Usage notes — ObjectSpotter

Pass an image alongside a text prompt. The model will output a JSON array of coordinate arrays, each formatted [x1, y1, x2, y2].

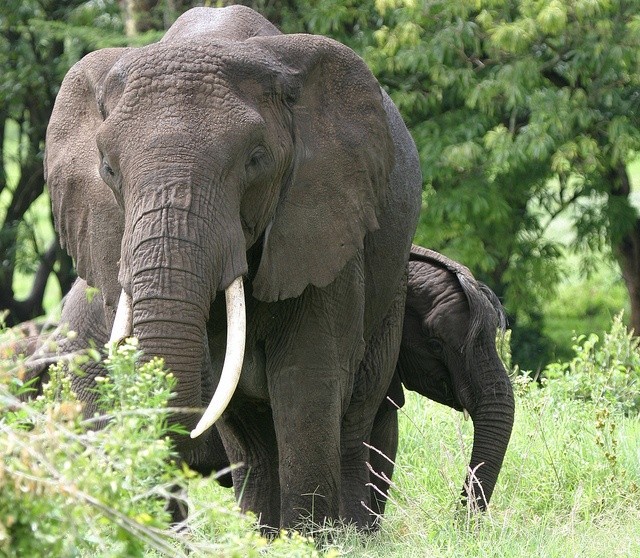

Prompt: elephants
[[7, 242, 516, 527], [42, 3, 424, 558]]
